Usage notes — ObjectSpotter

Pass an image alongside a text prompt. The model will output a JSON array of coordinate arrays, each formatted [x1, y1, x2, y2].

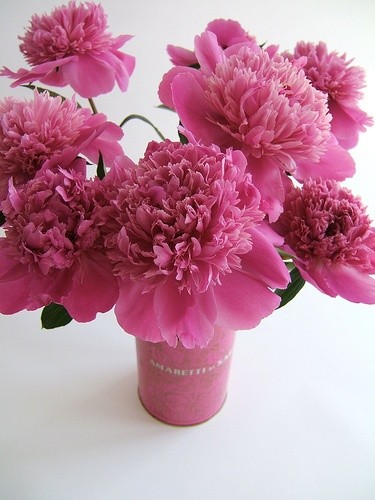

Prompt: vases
[[136, 325, 236, 426]]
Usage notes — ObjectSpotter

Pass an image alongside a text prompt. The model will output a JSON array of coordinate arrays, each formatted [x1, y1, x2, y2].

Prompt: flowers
[[0, 0, 374, 351]]
